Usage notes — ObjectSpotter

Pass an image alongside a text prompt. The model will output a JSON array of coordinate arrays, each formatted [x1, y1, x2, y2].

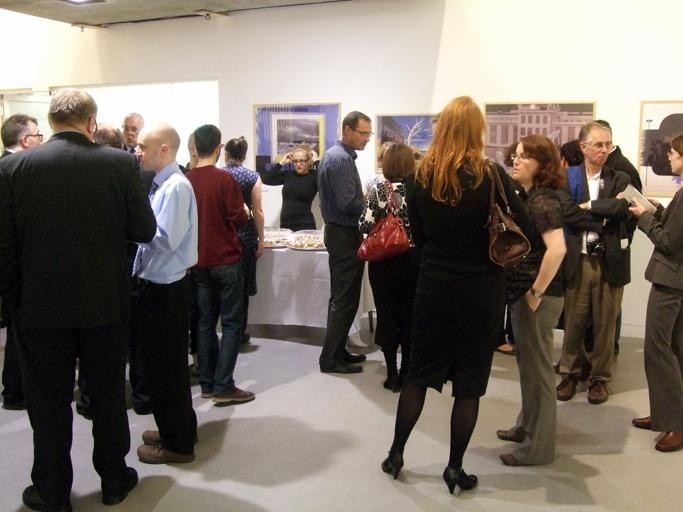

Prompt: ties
[[125, 146, 131, 153], [131, 183, 157, 278]]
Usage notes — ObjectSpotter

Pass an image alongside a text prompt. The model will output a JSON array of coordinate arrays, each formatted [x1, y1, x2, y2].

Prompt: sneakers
[[239, 334, 250, 344], [136, 444, 193, 463], [496, 342, 517, 356], [141, 431, 197, 445], [212, 387, 255, 402], [496, 430, 515, 441], [200, 382, 213, 398], [498, 453, 523, 465]]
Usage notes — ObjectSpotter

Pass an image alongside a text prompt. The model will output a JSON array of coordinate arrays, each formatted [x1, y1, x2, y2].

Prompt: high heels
[[442, 466, 477, 493], [381, 452, 403, 479], [383, 375, 401, 392]]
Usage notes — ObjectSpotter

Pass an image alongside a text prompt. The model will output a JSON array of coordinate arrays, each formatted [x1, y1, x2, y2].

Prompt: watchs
[[530, 287, 545, 299]]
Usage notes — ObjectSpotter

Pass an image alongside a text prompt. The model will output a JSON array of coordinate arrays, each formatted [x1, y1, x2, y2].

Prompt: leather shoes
[[631, 416, 652, 429], [318, 344, 362, 373], [655, 431, 683, 451], [21, 484, 72, 511], [555, 376, 576, 399], [76, 401, 94, 420], [101, 467, 137, 505], [343, 349, 366, 363], [587, 377, 613, 403]]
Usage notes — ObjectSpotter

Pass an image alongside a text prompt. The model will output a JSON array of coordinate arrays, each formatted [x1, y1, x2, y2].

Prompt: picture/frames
[[252, 103, 343, 173], [375, 113, 445, 174], [638, 100, 683, 198], [483, 102, 596, 175]]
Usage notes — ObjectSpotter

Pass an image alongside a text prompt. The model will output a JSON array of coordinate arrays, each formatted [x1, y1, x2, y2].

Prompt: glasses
[[24, 134, 42, 142], [353, 129, 375, 138], [509, 154, 541, 162], [582, 143, 616, 152], [120, 125, 138, 132], [290, 158, 308, 163], [666, 152, 678, 161], [88, 116, 97, 135]]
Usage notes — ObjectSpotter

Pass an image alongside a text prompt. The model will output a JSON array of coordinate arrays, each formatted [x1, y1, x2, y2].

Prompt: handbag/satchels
[[356, 213, 409, 262], [483, 200, 531, 271]]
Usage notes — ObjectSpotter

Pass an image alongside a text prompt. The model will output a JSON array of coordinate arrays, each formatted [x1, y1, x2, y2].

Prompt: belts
[[136, 278, 183, 287]]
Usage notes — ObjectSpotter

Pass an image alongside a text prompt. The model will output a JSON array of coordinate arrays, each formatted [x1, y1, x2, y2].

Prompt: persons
[[584, 119, 643, 363], [260, 144, 322, 231], [378, 93, 539, 494], [367, 141, 418, 394], [556, 120, 632, 404], [2, 89, 158, 509], [496, 141, 520, 354], [558, 139, 584, 168], [314, 109, 373, 374], [366, 142, 396, 190], [627, 130, 683, 453], [2, 113, 265, 464], [496, 135, 568, 465]]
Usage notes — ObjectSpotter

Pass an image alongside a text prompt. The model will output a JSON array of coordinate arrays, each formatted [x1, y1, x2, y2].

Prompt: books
[[621, 183, 658, 219]]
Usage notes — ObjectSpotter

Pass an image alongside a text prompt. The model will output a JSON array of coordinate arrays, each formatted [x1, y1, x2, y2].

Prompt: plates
[[286, 235, 328, 251]]
